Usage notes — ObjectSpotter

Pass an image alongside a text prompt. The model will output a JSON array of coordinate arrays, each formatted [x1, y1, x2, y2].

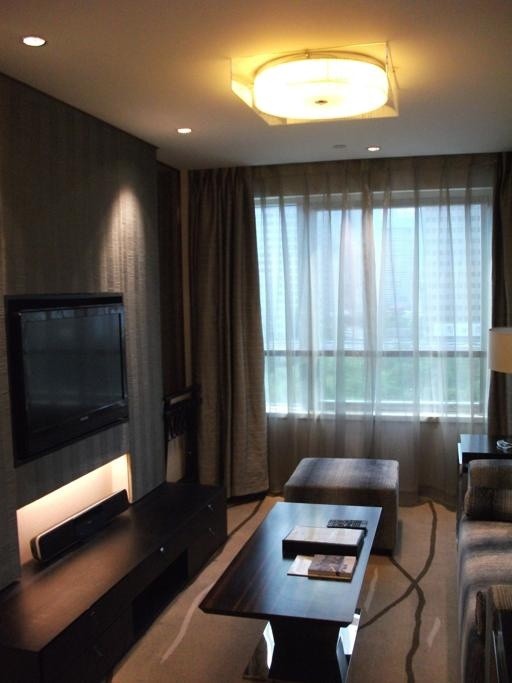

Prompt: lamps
[[487, 326, 512, 454], [231, 40, 399, 126]]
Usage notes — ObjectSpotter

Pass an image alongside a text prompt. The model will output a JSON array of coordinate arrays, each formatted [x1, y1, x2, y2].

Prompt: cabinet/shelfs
[[1, 482, 227, 682]]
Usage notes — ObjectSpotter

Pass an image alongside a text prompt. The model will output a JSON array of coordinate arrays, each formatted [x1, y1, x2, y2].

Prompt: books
[[306, 553, 357, 578], [286, 552, 358, 581]]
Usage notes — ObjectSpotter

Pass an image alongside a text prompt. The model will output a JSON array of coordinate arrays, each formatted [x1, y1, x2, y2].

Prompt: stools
[[283, 457, 399, 557]]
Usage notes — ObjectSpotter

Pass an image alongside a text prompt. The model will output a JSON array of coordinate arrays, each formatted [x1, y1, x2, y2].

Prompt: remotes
[[327, 519, 368, 528]]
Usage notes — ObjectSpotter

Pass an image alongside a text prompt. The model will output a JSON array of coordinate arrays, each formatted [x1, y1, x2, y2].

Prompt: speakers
[[29, 488, 130, 562]]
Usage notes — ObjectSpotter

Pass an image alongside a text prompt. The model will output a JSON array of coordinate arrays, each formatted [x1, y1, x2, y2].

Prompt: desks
[[457, 434, 511, 476]]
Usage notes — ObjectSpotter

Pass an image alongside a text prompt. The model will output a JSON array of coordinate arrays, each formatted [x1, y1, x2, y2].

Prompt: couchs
[[456, 458, 511, 682]]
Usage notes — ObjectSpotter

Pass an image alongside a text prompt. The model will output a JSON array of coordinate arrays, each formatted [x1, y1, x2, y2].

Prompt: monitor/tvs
[[4, 292, 131, 468]]
